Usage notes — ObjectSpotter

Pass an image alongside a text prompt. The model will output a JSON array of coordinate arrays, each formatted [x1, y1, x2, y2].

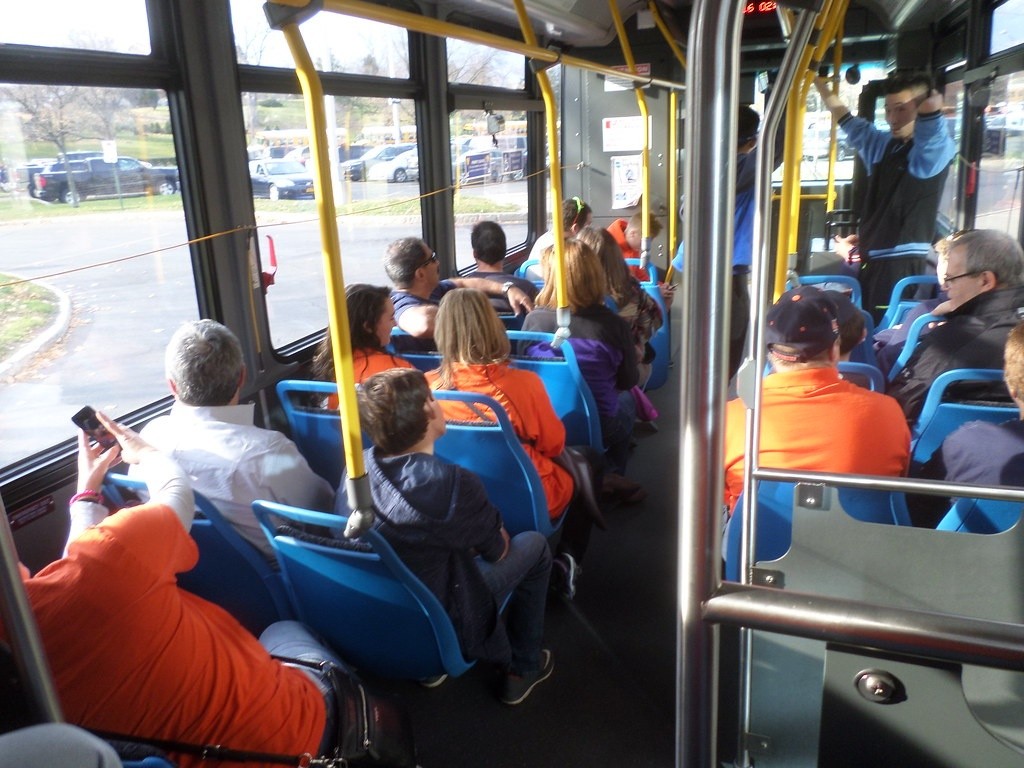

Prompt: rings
[[520, 297, 526, 303]]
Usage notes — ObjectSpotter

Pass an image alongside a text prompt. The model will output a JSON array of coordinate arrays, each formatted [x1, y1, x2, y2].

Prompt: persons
[[831, 235, 862, 277], [125, 320, 335, 573], [425, 288, 605, 601], [721, 284, 911, 569], [308, 283, 416, 408], [875, 229, 1024, 531], [517, 237, 648, 502], [16, 412, 354, 767], [825, 291, 867, 361], [468, 221, 538, 300], [329, 367, 574, 705], [729, 71, 787, 375], [384, 238, 534, 338], [811, 70, 955, 324], [529, 194, 677, 432]]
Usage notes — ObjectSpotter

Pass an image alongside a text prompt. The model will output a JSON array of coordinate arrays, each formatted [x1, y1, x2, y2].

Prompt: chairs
[[0, 259, 1024, 767]]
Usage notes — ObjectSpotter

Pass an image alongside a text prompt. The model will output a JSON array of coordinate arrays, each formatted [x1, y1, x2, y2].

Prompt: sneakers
[[502, 649, 554, 706]]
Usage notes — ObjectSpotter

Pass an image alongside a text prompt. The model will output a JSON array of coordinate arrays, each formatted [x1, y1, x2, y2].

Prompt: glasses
[[935, 270, 1000, 293], [573, 196, 587, 226], [420, 251, 439, 269]]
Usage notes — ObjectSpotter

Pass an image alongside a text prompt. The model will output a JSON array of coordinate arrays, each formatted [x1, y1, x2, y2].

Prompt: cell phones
[[71, 406, 123, 457]]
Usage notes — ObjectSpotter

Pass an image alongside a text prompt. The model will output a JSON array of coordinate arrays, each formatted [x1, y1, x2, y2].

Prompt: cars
[[804, 122, 856, 162], [0, 133, 529, 209]]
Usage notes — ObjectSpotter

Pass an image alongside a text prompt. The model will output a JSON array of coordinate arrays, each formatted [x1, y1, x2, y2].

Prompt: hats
[[825, 290, 863, 357], [768, 286, 843, 362]]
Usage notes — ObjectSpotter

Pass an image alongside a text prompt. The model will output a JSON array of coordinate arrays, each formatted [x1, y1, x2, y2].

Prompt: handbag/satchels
[[320, 660, 416, 768], [630, 384, 660, 422]]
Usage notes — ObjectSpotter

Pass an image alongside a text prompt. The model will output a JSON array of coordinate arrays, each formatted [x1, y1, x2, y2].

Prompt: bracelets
[[69, 490, 104, 503], [502, 282, 515, 298]]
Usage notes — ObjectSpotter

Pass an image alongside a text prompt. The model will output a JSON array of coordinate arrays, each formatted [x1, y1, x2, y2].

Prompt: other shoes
[[602, 473, 640, 499], [574, 564, 583, 577], [614, 491, 650, 506], [553, 552, 576, 602], [633, 417, 658, 434], [421, 673, 448, 688]]
